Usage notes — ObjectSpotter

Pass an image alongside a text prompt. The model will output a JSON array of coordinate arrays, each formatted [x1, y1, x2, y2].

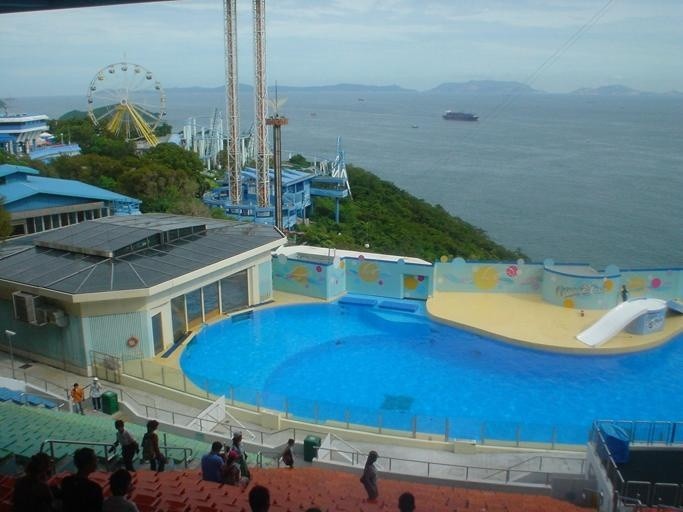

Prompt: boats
[[442, 109, 480, 121]]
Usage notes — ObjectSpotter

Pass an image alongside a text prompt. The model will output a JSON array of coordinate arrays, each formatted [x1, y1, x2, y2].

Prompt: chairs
[[0, 386, 682, 512]]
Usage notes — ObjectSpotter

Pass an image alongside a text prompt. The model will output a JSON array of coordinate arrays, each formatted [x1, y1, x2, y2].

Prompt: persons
[[399, 492, 415, 511], [621, 285, 629, 302], [363, 451, 378, 499], [112, 420, 138, 473], [13, 451, 53, 511], [89, 377, 103, 411], [71, 382, 85, 414], [249, 485, 270, 512], [103, 469, 139, 511], [142, 420, 165, 471], [61, 447, 104, 511], [201, 431, 250, 492], [283, 438, 295, 467]]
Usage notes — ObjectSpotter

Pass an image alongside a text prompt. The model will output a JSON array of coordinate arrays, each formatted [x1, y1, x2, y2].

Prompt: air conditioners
[[11, 291, 69, 328]]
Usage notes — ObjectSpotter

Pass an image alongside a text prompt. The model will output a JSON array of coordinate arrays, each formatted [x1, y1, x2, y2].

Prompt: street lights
[[263, 82, 290, 233], [6, 329, 16, 380], [60, 133, 63, 144]]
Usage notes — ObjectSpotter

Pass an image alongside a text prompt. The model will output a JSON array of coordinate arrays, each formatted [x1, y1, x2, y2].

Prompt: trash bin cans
[[303, 435, 321, 462], [101, 391, 118, 415]]
